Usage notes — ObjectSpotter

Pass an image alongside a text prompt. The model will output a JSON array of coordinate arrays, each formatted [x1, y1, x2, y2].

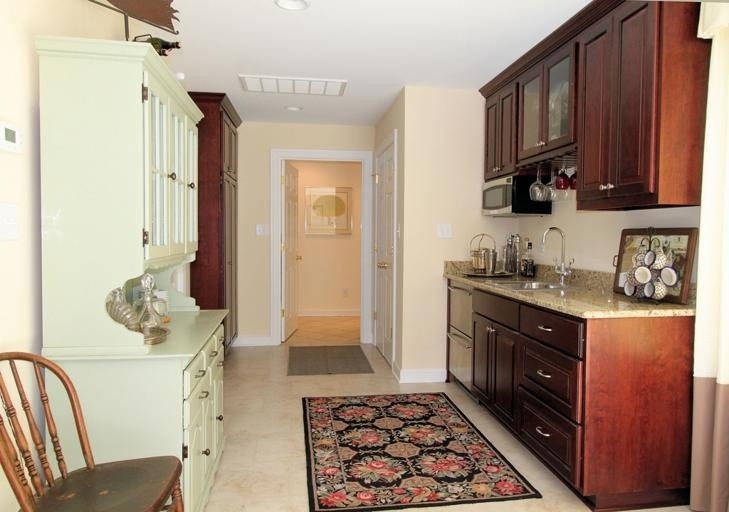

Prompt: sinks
[[491, 280, 565, 292]]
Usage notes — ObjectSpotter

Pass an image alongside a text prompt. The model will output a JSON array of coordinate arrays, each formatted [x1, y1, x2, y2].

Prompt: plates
[[463, 271, 514, 278]]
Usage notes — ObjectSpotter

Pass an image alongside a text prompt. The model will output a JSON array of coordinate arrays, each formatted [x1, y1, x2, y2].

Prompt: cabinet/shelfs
[[519, 294, 694, 512], [480, 60, 518, 183], [186, 93, 242, 352], [39, 32, 231, 512], [517, 13, 578, 170], [575, 1, 712, 211], [472, 287, 519, 443]]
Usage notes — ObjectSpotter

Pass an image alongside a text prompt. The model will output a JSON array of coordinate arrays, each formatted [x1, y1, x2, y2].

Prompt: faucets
[[542, 226, 575, 286]]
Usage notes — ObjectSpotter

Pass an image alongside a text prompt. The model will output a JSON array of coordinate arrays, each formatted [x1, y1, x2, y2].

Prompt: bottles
[[106, 288, 138, 333], [522, 238, 529, 275], [514, 234, 521, 274], [138, 272, 163, 338], [506, 234, 512, 272], [526, 242, 535, 277]]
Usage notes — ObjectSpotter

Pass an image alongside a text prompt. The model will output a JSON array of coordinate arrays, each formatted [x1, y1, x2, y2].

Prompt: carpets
[[301, 391, 542, 512], [287, 345, 374, 378]]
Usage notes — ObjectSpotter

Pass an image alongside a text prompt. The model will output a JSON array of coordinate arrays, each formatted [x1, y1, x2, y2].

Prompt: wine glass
[[556, 162, 571, 189], [555, 159, 568, 202], [568, 162, 578, 189], [547, 163, 558, 201], [529, 162, 548, 203]]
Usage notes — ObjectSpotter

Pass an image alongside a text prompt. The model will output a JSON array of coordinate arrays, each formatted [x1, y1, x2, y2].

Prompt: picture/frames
[[303, 185, 355, 235], [612, 223, 698, 306]]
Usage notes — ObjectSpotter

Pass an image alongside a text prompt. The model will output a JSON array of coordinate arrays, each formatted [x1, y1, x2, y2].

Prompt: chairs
[[0, 353, 186, 512]]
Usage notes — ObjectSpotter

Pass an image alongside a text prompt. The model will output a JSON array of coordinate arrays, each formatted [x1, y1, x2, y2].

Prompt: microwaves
[[481, 175, 552, 215]]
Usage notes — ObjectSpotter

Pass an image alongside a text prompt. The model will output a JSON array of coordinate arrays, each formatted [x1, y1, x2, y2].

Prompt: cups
[[626, 266, 652, 287], [631, 247, 646, 268], [655, 266, 678, 286], [483, 249, 497, 274], [624, 280, 644, 299], [644, 246, 667, 270], [644, 277, 667, 300]]
